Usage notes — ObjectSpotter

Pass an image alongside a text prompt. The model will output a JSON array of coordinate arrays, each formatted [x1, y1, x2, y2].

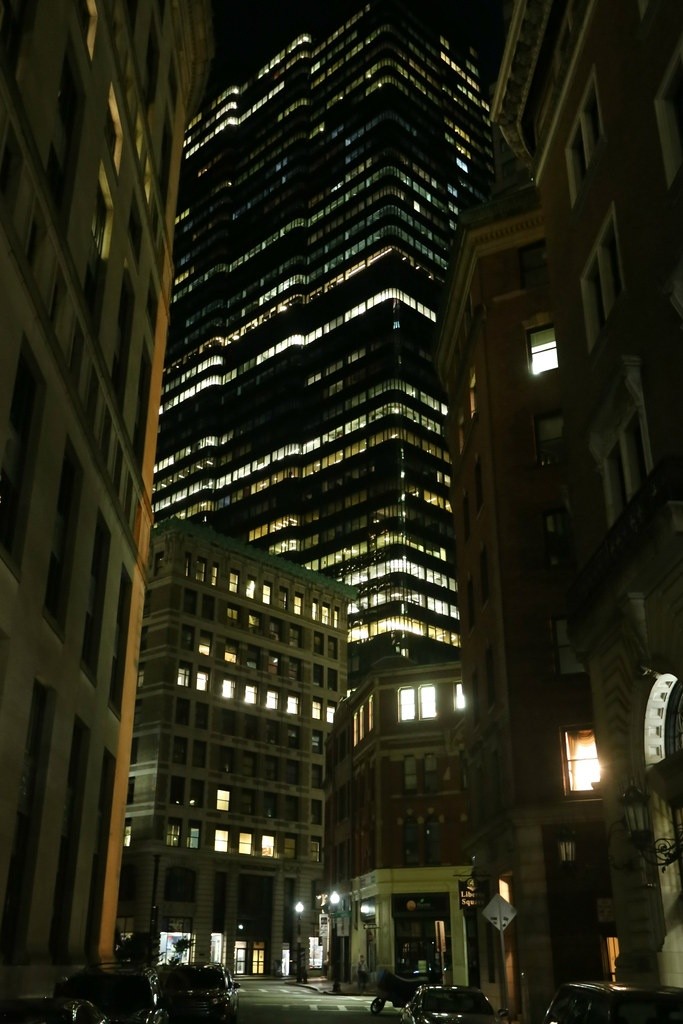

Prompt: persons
[[355, 953, 368, 995]]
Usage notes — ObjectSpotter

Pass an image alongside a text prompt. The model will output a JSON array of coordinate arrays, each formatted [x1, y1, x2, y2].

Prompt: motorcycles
[[372, 970, 431, 1012]]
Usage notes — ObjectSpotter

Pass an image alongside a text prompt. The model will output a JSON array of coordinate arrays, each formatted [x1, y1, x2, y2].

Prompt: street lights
[[328, 890, 345, 991], [295, 900, 304, 982]]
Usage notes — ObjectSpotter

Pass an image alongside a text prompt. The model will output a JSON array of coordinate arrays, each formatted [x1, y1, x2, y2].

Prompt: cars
[[0, 995, 111, 1024], [399, 984, 509, 1024]]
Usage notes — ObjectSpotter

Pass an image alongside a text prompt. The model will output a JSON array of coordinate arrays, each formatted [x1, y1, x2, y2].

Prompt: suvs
[[55, 961, 169, 1024], [164, 961, 241, 1024], [541, 982, 683, 1024]]
